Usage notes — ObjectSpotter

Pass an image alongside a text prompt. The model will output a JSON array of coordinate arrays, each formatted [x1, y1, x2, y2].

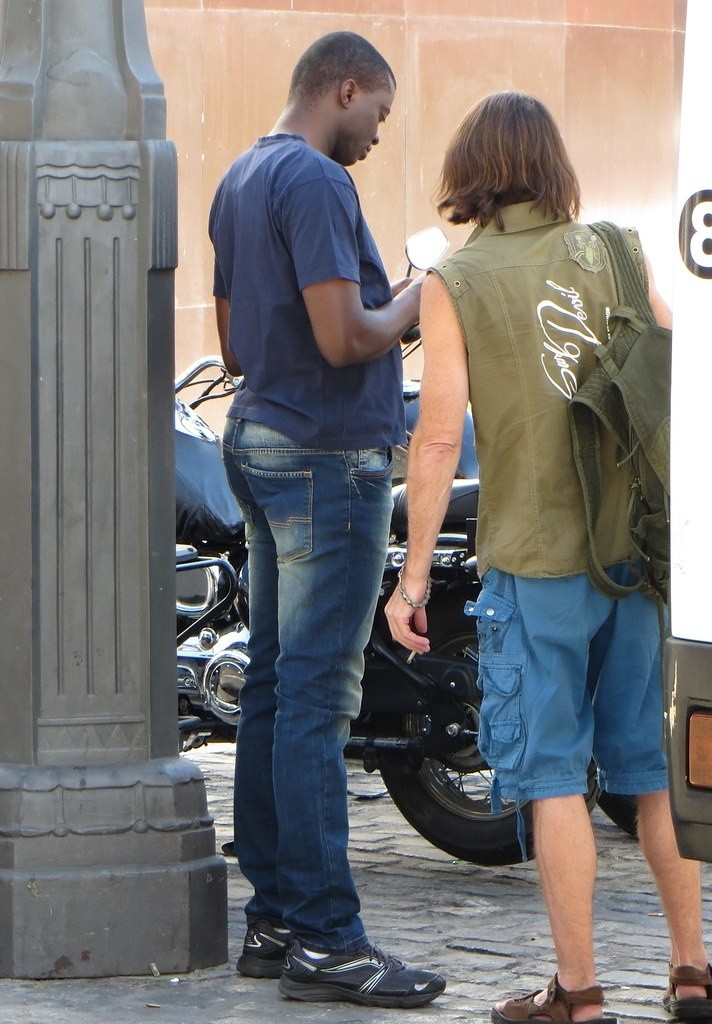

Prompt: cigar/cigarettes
[[407, 649, 416, 664]]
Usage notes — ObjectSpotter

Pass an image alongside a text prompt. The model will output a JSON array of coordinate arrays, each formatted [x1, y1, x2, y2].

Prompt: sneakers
[[236, 920, 296, 977], [279, 938, 446, 1008]]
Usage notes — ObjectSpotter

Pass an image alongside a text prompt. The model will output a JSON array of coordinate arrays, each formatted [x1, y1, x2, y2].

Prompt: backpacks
[[568, 220, 673, 750]]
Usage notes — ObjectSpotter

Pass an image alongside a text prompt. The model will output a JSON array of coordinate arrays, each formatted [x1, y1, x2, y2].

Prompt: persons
[[385, 92, 711, 1023], [208, 27, 448, 1007]]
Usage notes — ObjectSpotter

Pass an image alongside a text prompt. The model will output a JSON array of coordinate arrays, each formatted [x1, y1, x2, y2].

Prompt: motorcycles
[[172, 225, 640, 866]]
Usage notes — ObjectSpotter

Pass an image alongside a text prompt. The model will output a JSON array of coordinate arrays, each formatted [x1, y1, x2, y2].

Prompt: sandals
[[491, 972, 618, 1024], [662, 962, 712, 1018]]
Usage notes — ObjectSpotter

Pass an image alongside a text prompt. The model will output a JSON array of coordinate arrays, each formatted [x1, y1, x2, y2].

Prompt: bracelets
[[397, 565, 433, 608]]
